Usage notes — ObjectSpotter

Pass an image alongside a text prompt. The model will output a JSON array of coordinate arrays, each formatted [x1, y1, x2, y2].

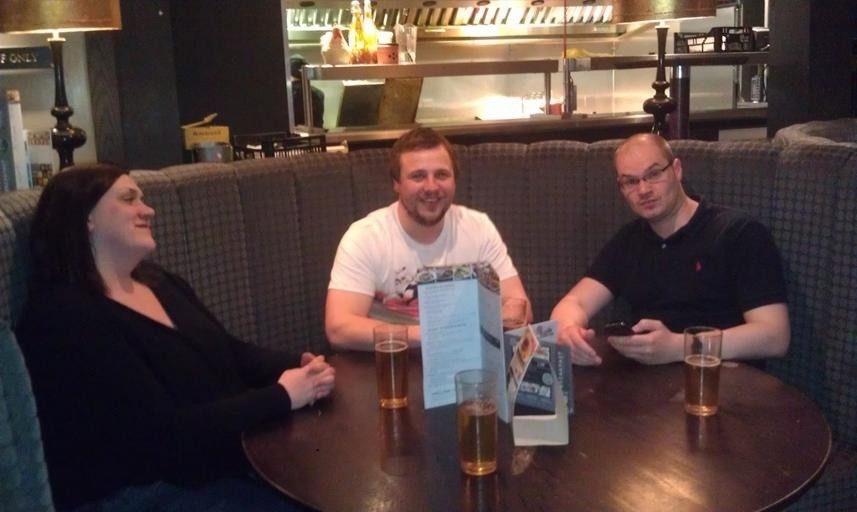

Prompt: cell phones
[[602, 321, 632, 338]]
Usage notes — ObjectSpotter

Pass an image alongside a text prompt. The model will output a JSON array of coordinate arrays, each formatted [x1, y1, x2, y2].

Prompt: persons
[[320, 125, 535, 353], [25, 163, 338, 511], [548, 131, 792, 367], [289, 53, 324, 127]]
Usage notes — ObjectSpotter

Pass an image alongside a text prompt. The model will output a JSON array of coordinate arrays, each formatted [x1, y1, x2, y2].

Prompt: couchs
[[0, 141, 849, 511]]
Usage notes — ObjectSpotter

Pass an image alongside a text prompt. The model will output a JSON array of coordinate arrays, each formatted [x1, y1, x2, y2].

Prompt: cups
[[682, 325, 723, 417], [372, 322, 409, 409], [455, 368, 499, 476], [394, 23, 418, 63]]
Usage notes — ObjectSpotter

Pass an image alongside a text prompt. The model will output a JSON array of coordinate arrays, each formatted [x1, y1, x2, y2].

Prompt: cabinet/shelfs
[[297, 57, 782, 150]]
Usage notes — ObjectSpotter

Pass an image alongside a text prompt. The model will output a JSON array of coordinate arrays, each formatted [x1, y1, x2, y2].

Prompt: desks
[[239, 329, 834, 512]]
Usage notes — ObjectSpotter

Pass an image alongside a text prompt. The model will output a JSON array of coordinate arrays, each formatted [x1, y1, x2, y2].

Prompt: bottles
[[347, 0, 379, 62]]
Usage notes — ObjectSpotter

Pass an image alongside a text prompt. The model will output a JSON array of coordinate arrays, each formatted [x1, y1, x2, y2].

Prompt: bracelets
[[689, 332, 702, 355]]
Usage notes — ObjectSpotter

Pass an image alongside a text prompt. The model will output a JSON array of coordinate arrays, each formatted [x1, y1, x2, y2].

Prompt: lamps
[[0, 0, 122, 169]]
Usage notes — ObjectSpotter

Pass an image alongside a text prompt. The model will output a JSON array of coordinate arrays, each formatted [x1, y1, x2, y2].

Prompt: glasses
[[616, 161, 673, 191]]
